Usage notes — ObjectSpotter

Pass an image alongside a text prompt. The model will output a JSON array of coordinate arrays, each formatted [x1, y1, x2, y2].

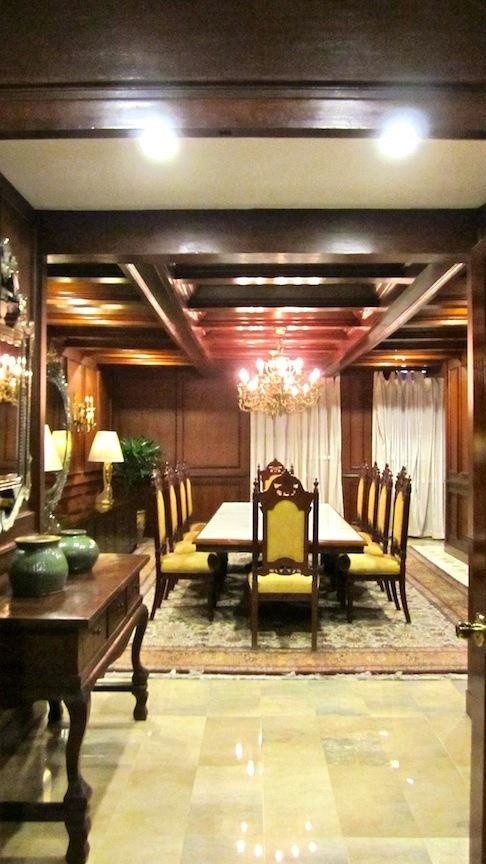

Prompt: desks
[[0, 553, 149, 864]]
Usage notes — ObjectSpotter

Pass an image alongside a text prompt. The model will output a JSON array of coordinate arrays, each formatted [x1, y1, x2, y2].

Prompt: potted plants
[[112, 434, 161, 541]]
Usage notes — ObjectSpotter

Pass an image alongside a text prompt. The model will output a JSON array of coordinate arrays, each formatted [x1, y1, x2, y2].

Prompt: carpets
[[105, 538, 469, 673]]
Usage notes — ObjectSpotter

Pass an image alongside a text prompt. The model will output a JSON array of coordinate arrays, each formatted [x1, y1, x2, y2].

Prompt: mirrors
[[0, 237, 35, 531], [45, 342, 72, 532]]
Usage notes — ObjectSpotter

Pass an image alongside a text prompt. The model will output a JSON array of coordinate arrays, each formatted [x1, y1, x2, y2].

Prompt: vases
[[7, 535, 69, 598], [58, 528, 99, 573]]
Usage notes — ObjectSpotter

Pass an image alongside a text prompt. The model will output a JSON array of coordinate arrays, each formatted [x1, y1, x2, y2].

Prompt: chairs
[[352, 461, 380, 539], [248, 478, 326, 650], [336, 466, 410, 624], [150, 461, 221, 624], [257, 460, 295, 497], [335, 464, 394, 599]]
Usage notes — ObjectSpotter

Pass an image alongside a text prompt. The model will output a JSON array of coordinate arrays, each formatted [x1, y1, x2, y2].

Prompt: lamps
[[87, 430, 124, 505], [73, 396, 96, 433], [44, 424, 63, 472], [0, 353, 32, 407], [51, 430, 71, 466], [236, 330, 322, 424]]
